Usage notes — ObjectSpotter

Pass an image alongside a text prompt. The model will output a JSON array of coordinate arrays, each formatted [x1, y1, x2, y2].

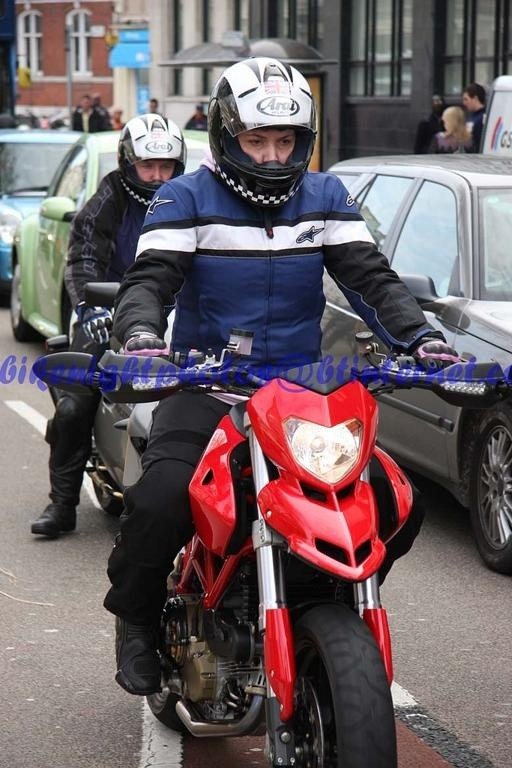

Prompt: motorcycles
[[33, 328, 512, 765], [42, 280, 177, 522]]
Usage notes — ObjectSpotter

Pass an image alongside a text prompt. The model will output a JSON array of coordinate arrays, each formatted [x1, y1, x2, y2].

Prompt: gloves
[[412, 337, 460, 374], [123, 323, 170, 356], [78, 304, 113, 344]]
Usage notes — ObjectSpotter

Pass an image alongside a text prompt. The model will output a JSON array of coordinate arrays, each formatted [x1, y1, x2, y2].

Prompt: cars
[[7, 124, 213, 355], [314, 148, 511, 577], [1, 125, 87, 304]]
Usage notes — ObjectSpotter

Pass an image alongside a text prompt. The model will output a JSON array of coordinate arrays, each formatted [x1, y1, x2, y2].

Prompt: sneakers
[[116, 614, 160, 692], [32, 502, 75, 533]]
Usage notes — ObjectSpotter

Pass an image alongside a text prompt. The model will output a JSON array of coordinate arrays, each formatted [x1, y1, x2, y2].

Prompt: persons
[[1, 94, 210, 132], [31, 113, 187, 539], [462, 83, 488, 154], [103, 56, 461, 696], [427, 106, 474, 154]]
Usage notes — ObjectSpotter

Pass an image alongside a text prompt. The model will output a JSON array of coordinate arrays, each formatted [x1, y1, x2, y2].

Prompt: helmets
[[207, 55, 316, 209], [118, 113, 187, 209]]
